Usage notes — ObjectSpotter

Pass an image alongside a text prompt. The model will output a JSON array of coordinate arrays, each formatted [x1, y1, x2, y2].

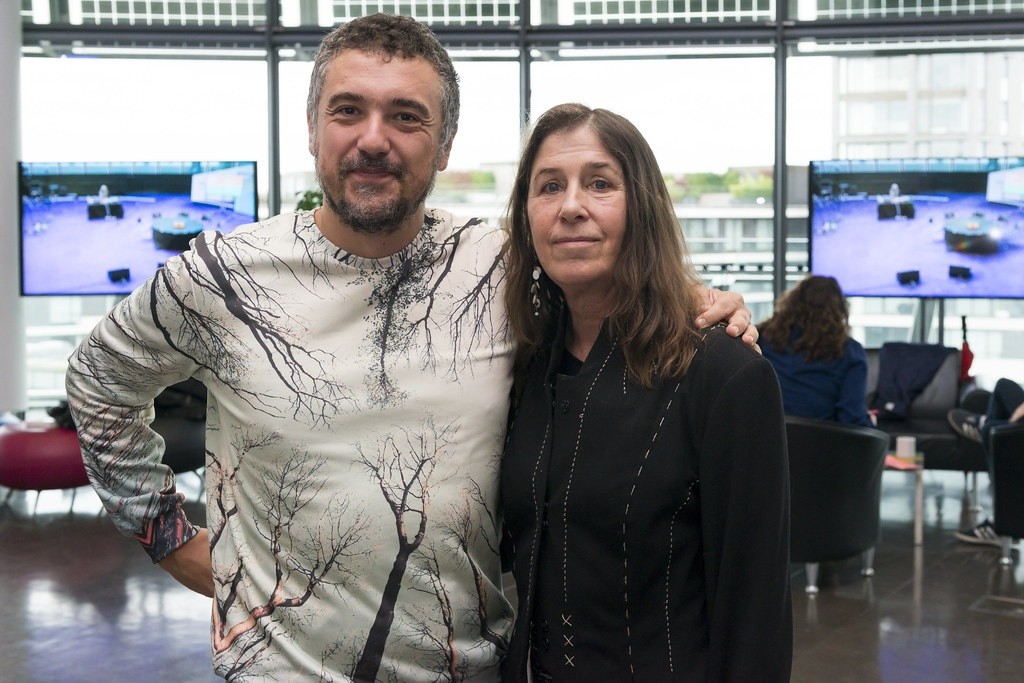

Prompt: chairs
[[863, 347, 1024, 547], [787, 414, 891, 592]]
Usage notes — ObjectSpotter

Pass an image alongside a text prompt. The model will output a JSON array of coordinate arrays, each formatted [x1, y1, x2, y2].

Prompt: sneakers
[[954, 519, 1020, 548], [947, 408, 989, 444]]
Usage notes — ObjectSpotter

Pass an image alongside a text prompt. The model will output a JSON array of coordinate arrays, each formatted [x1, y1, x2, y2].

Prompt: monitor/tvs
[[18, 161, 258, 297], [808, 156, 1024, 300]]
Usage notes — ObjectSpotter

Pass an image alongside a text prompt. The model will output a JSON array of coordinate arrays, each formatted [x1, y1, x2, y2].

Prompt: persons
[[947, 378, 1024, 545], [65, 13, 762, 683], [500, 103, 793, 683], [756, 275, 875, 428]]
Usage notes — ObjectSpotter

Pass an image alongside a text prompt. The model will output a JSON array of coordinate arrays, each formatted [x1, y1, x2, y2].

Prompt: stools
[[0, 417, 207, 518]]
